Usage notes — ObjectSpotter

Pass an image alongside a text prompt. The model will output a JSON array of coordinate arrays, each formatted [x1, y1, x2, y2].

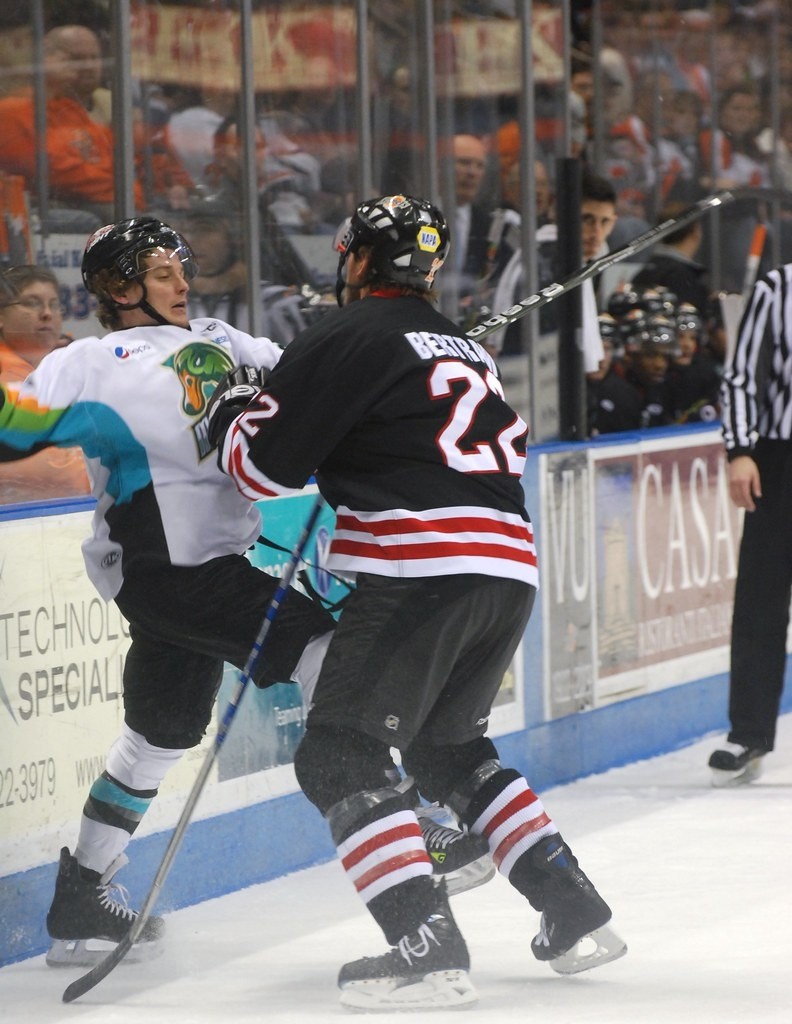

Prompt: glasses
[[0, 298, 67, 316]]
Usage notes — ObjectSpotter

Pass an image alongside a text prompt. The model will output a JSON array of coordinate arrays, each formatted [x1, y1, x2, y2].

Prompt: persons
[[488, 178, 619, 373], [4, 216, 333, 964], [7, 20, 189, 219], [452, 132, 495, 273], [703, 257, 790, 786], [493, 4, 791, 434], [209, 196, 628, 1006], [4, 260, 68, 406]]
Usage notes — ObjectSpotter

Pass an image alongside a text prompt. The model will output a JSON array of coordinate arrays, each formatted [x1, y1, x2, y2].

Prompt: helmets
[[350, 194, 450, 289], [593, 282, 702, 354], [82, 216, 170, 294]]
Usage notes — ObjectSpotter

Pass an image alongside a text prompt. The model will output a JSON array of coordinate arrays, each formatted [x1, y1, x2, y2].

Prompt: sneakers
[[506, 834, 628, 975], [709, 732, 774, 788], [46, 846, 166, 962], [337, 874, 478, 1008], [394, 776, 496, 896]]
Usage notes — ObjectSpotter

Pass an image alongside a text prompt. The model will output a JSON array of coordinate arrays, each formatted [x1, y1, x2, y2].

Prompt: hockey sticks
[[467, 190, 734, 345], [60, 491, 328, 1004]]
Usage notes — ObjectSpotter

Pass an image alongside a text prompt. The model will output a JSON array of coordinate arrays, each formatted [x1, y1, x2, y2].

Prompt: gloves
[[206, 363, 272, 476]]
